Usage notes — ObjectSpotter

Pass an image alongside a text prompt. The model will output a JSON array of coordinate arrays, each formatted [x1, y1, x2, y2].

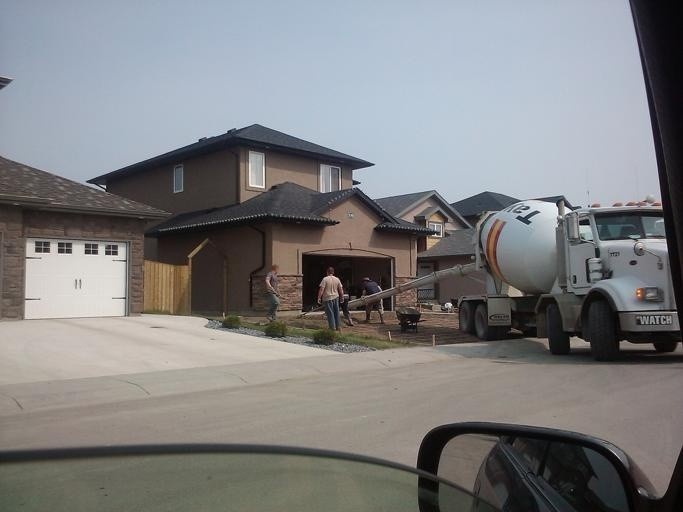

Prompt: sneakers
[[267, 315, 276, 322], [327, 326, 342, 331]]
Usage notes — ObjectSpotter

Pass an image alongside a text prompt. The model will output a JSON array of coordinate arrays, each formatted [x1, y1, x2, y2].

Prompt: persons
[[318, 267, 345, 333], [334, 272, 354, 326], [360, 275, 385, 323], [263, 264, 282, 322]]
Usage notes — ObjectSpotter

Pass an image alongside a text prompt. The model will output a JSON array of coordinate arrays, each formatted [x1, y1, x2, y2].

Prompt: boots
[[363, 314, 371, 323], [347, 317, 354, 326], [380, 314, 386, 323]]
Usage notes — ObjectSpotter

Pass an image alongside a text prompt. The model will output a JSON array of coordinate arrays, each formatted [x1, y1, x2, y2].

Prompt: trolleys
[[395, 306, 420, 333]]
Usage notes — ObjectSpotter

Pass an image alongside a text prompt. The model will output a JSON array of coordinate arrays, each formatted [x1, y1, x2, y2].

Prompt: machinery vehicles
[[456, 197, 681, 362]]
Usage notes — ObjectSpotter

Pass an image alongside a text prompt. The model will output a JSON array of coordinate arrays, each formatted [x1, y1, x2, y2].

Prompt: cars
[[471, 434, 630, 511]]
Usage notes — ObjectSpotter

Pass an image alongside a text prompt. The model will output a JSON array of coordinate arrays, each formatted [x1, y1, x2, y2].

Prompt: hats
[[360, 277, 373, 282]]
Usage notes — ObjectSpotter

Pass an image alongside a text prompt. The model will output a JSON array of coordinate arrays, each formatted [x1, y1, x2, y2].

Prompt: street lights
[[0, 75, 14, 90]]
